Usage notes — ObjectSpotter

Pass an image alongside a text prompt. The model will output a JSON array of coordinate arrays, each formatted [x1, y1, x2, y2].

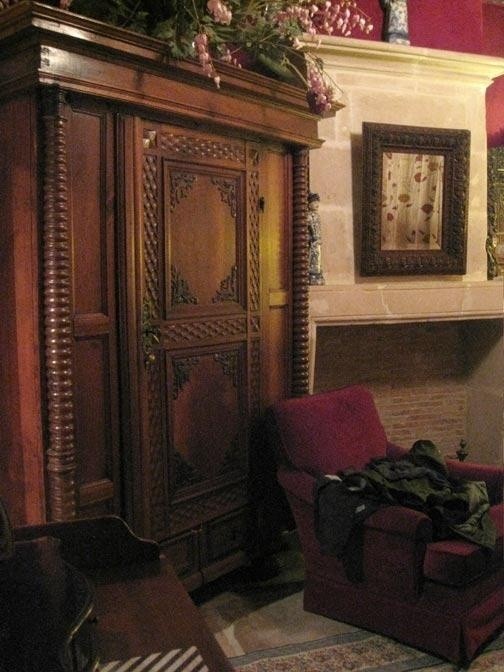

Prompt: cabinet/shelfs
[[0, 0, 348, 592]]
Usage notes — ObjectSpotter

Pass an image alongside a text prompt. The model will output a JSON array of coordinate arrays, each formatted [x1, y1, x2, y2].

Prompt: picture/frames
[[361, 120, 470, 276]]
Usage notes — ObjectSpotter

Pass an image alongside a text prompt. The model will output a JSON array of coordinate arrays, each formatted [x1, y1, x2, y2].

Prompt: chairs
[[274, 440, 504, 670]]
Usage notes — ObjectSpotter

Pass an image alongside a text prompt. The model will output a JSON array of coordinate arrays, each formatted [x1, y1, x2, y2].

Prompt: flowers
[[57, 0, 374, 117]]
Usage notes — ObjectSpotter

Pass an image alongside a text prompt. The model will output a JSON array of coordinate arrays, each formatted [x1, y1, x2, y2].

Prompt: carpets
[[226, 622, 504, 672]]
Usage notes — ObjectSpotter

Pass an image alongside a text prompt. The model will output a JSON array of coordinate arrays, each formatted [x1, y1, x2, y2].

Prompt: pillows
[[276, 385, 387, 476]]
